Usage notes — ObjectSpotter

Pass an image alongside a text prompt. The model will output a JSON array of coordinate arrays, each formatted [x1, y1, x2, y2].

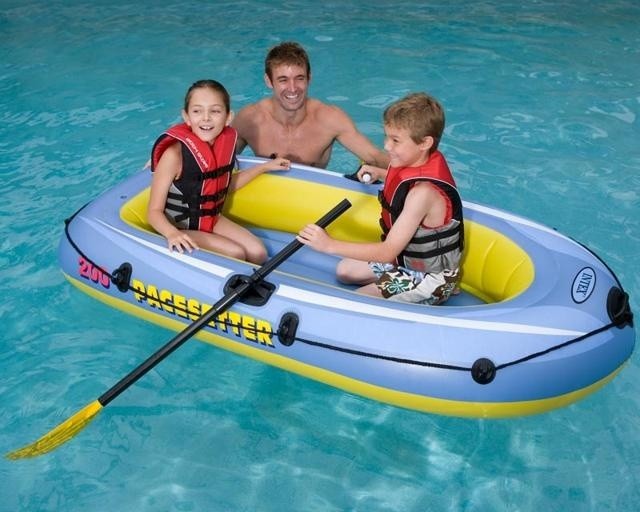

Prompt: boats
[[57, 155, 636, 418]]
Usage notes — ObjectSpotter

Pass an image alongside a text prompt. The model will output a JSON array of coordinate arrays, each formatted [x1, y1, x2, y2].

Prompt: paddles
[[6, 198, 351, 462]]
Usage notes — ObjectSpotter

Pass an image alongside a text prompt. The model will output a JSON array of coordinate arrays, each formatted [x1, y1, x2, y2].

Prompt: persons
[[295, 90, 465, 308], [227, 38, 393, 176], [147, 79, 291, 269]]
